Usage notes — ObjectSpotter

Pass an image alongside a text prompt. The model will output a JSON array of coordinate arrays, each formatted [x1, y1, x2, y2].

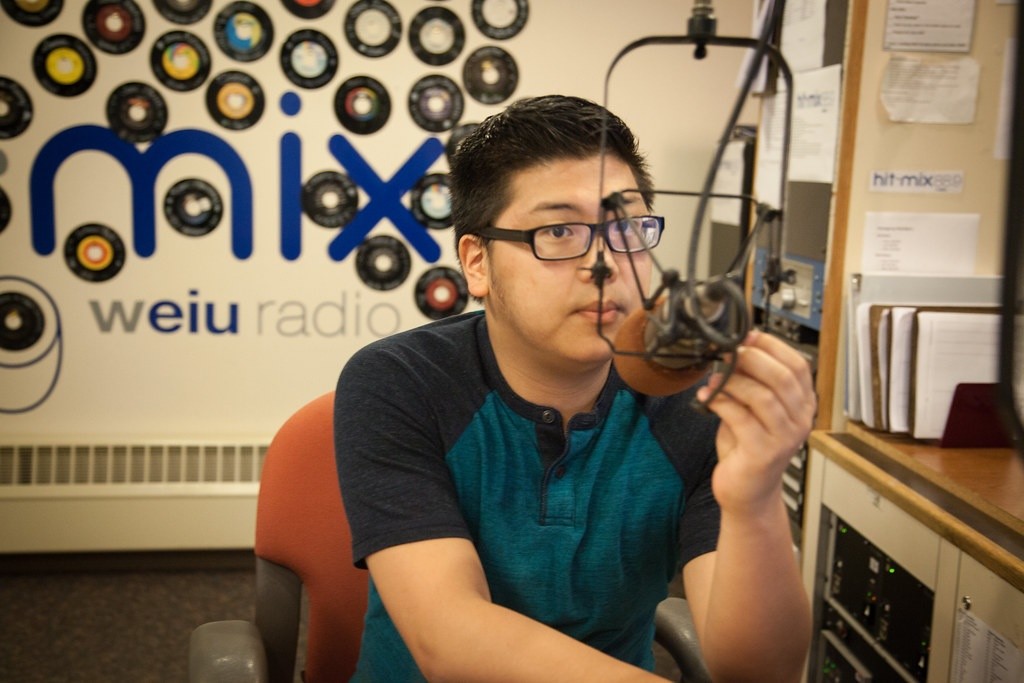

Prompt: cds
[[0, 0, 529, 353]]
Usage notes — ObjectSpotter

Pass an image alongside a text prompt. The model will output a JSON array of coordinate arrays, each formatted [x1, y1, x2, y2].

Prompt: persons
[[333, 94, 816, 683]]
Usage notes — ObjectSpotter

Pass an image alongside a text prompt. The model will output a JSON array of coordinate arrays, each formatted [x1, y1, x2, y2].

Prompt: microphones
[[613, 275, 732, 397]]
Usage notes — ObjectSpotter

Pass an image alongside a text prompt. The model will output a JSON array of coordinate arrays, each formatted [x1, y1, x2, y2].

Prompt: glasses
[[471, 215, 665, 261]]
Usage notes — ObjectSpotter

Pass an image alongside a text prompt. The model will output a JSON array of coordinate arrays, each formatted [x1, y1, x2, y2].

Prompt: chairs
[[188, 386, 381, 683]]
